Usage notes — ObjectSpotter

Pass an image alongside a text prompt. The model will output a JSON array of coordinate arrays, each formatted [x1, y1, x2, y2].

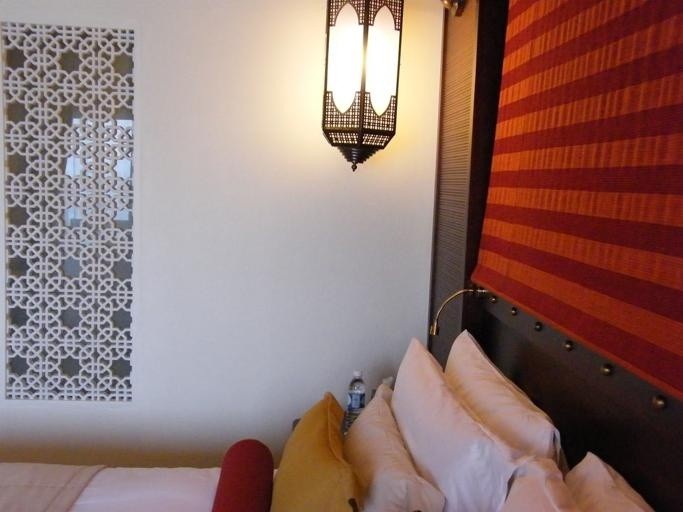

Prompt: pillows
[[496, 454, 581, 511], [444, 328, 565, 463], [564, 449, 656, 511], [338, 378, 446, 511], [268, 390, 369, 512], [210, 439, 275, 512], [391, 336, 538, 511]]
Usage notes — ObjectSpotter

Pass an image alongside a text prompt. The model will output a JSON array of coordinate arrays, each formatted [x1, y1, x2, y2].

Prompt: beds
[[0, 326, 683, 512]]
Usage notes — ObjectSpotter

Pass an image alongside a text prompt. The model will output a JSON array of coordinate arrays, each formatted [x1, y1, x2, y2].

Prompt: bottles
[[345, 370, 366, 429]]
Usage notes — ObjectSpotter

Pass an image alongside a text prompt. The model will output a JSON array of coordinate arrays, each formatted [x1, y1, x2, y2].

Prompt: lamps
[[319, 0, 466, 173], [429, 286, 487, 335]]
